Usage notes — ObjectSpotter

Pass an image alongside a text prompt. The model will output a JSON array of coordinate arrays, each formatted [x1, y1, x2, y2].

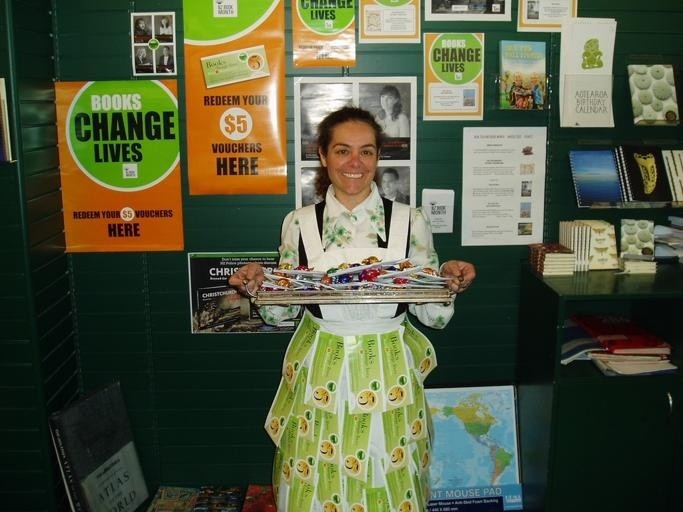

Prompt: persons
[[134, 47, 150, 66], [159, 16, 172, 36], [373, 86, 409, 138], [133, 17, 146, 37], [226, 107, 477, 512], [378, 168, 409, 205], [158, 45, 174, 73]]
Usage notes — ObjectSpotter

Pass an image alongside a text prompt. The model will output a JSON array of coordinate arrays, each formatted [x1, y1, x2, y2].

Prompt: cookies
[[628, 65, 679, 125], [621, 219, 653, 256]]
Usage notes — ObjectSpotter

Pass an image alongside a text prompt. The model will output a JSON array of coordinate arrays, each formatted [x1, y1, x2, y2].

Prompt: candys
[[265, 256, 438, 291]]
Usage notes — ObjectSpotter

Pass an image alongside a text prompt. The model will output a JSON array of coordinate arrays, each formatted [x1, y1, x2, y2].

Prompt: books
[[567, 148, 683, 210], [525, 216, 683, 277], [556, 313, 678, 376]]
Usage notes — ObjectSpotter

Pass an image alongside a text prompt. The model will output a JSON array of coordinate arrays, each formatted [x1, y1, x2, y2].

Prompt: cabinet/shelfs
[[517, 260, 681, 512]]
